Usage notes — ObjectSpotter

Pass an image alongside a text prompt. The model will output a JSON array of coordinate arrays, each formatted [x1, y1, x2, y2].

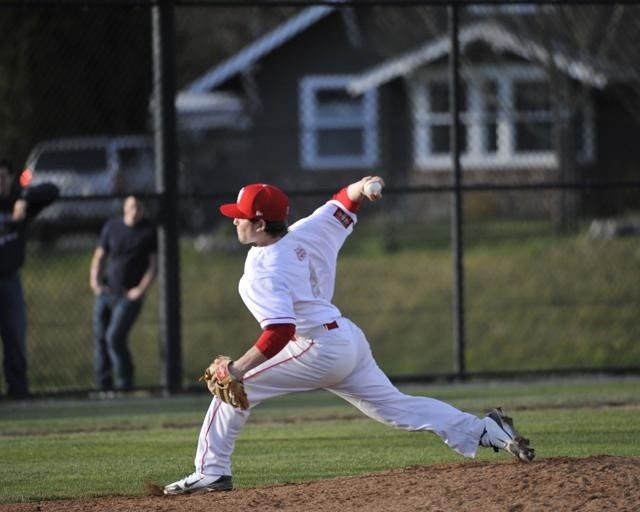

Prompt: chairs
[[479, 406, 536, 463], [164, 472, 232, 495]]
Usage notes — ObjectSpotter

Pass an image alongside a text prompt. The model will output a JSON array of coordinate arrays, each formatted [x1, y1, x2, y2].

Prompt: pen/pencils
[[21, 138, 199, 251]]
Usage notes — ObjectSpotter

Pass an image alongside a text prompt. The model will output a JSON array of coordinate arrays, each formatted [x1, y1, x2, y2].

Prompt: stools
[[291, 320, 338, 341]]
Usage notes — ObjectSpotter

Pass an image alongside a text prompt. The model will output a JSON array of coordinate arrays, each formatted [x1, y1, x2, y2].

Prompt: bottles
[[220, 183, 290, 222]]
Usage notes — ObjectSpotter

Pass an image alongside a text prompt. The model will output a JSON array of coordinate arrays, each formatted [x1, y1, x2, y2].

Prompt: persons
[[85, 192, 158, 399], [0, 157, 35, 399], [157, 175, 536, 494]]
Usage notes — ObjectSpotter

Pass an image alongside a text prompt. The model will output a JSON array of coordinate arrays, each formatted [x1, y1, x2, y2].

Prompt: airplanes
[[205, 356, 251, 413]]
